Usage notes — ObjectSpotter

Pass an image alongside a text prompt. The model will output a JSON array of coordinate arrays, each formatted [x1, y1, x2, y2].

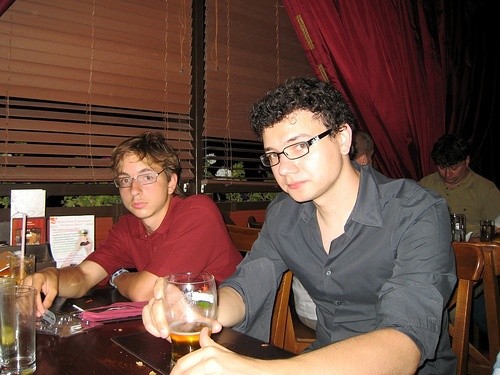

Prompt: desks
[[0, 269, 299, 375]]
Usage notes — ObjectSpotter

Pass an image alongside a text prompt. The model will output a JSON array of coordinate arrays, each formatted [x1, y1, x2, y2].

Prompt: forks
[[72, 304, 121, 312]]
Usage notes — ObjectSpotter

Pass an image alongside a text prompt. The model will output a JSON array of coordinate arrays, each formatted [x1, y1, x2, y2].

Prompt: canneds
[[450, 214, 466, 242]]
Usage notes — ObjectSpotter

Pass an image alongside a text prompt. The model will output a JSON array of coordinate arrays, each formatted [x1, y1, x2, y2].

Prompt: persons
[[17, 131, 243, 318], [141, 74, 457, 375], [417, 135, 500, 332], [292, 132, 374, 329]]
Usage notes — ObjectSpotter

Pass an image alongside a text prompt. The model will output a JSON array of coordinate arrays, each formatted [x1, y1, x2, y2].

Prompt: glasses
[[260, 128, 333, 168], [113, 167, 168, 188]]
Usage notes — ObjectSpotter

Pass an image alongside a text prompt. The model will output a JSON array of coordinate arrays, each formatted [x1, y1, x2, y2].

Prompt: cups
[[450, 213, 466, 243], [163, 271, 218, 372], [0, 285, 37, 375], [480, 218, 495, 242], [9, 252, 36, 283]]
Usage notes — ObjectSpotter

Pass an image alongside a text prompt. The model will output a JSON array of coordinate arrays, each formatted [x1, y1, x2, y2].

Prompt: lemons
[[1, 326, 15, 345]]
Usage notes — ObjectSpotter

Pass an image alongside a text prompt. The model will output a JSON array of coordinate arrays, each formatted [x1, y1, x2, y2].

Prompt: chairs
[[228, 228, 500, 375]]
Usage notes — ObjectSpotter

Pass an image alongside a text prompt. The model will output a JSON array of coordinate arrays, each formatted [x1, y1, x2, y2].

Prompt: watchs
[[110, 268, 129, 288]]
